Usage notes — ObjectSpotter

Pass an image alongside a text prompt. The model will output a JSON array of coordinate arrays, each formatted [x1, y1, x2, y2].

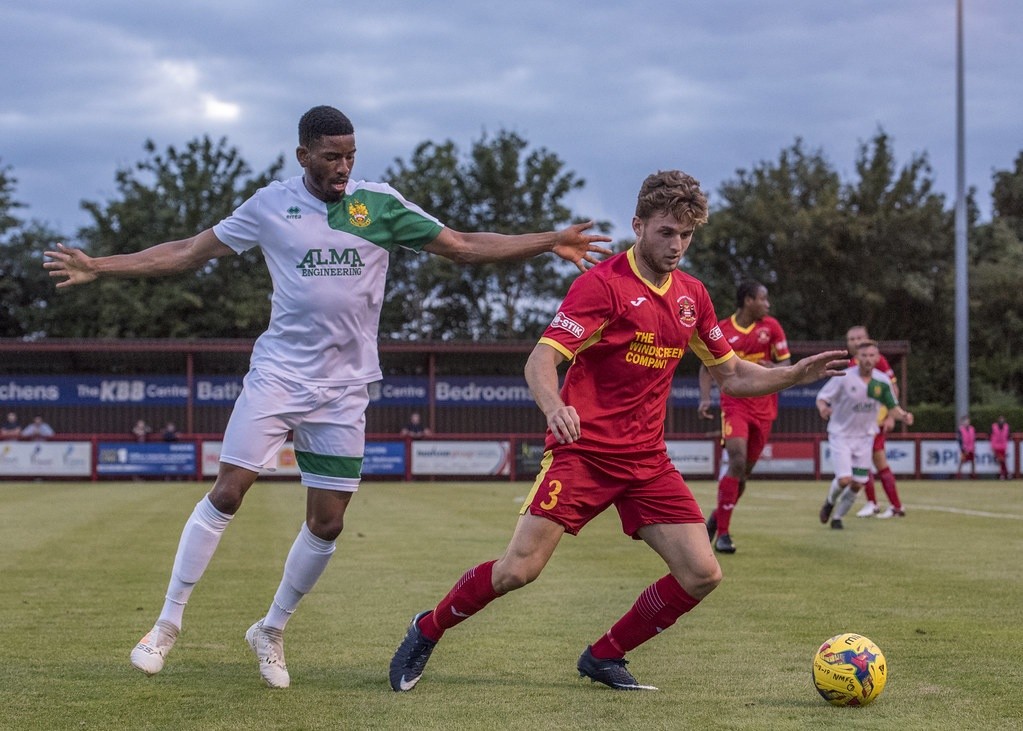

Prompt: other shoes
[[820, 498, 837, 523], [876, 505, 905, 519], [706, 514, 717, 543], [856, 500, 880, 518], [715, 535, 735, 553], [831, 519, 841, 529]]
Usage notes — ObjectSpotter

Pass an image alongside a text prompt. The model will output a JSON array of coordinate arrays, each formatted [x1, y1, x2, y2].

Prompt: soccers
[[812, 632, 888, 705]]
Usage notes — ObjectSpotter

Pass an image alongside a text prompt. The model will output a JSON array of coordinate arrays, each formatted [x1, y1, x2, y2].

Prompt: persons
[[23, 416, 53, 436], [956, 416, 977, 480], [43, 106, 612, 689], [164, 422, 180, 440], [133, 420, 151, 435], [402, 413, 430, 434], [697, 280, 792, 553], [0, 413, 21, 436], [989, 415, 1012, 480], [848, 324, 906, 520], [816, 341, 913, 530], [388, 170, 851, 692]]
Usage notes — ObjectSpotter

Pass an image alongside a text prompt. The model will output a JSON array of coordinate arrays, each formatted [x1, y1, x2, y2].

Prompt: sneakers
[[576, 645, 657, 690], [244, 619, 291, 690], [390, 610, 439, 692], [130, 625, 175, 677]]
